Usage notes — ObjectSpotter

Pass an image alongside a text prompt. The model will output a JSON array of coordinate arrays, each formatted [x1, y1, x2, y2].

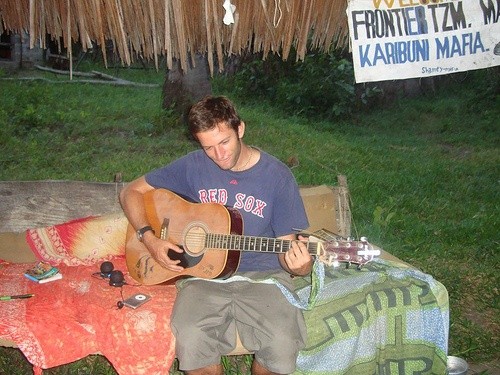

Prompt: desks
[[0, 173, 449, 375]]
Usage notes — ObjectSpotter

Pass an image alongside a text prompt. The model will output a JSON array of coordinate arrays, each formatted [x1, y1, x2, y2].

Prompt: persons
[[118, 97, 316, 375]]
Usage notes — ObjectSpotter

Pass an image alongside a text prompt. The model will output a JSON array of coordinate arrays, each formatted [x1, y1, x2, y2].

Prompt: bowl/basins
[[447, 356, 469, 375]]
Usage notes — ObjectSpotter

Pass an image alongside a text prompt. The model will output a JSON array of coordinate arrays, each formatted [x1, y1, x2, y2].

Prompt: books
[[22, 261, 63, 284]]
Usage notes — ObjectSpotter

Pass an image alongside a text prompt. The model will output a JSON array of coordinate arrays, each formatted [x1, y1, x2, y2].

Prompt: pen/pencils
[[0, 294, 35, 300]]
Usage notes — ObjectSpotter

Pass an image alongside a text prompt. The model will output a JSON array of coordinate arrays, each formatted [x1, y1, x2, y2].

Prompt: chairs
[[298, 175, 352, 238]]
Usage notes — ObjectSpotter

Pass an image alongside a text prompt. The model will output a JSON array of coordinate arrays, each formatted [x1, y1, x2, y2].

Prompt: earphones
[[117, 301, 123, 308]]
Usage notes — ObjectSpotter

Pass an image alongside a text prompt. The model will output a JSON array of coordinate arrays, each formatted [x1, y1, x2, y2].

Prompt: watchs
[[136, 225, 156, 242]]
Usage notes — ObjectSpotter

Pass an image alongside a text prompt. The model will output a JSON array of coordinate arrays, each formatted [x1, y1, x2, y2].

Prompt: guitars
[[124, 188, 381, 286]]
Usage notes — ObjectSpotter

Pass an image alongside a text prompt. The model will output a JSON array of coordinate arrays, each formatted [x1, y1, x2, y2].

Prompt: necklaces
[[232, 145, 253, 170]]
